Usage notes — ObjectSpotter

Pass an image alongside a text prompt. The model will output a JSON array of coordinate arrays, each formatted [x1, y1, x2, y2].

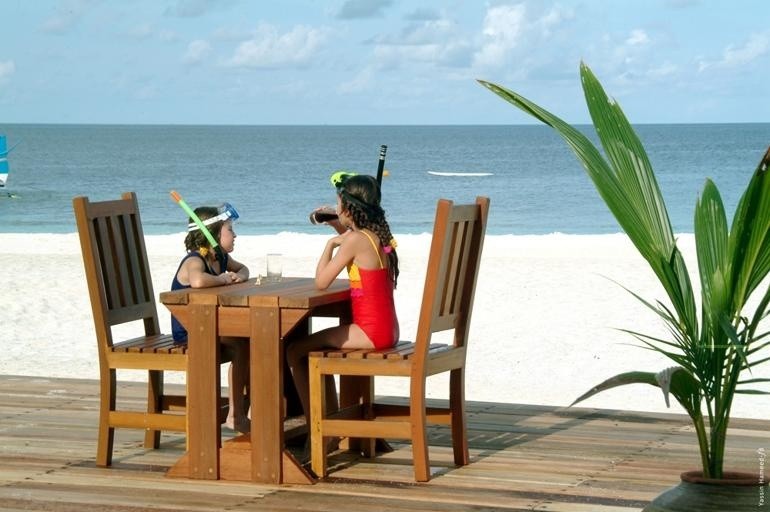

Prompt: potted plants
[[472, 59, 770, 512]]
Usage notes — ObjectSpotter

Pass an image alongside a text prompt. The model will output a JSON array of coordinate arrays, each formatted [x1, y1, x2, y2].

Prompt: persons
[[170, 207, 251, 436], [286, 175, 399, 459]]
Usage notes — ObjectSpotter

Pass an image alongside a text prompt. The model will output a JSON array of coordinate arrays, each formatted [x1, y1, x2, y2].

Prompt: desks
[[158, 273, 351, 486]]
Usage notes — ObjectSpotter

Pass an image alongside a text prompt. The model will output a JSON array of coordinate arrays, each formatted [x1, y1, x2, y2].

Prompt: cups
[[265, 249, 283, 283], [307, 201, 338, 223]]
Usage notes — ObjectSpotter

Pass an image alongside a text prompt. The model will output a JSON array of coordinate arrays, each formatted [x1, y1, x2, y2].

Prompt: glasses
[[330, 171, 372, 211], [187, 202, 239, 235]]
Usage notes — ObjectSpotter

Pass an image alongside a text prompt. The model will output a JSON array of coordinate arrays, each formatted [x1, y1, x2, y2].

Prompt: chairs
[[72, 189, 246, 465], [304, 195, 493, 484]]
